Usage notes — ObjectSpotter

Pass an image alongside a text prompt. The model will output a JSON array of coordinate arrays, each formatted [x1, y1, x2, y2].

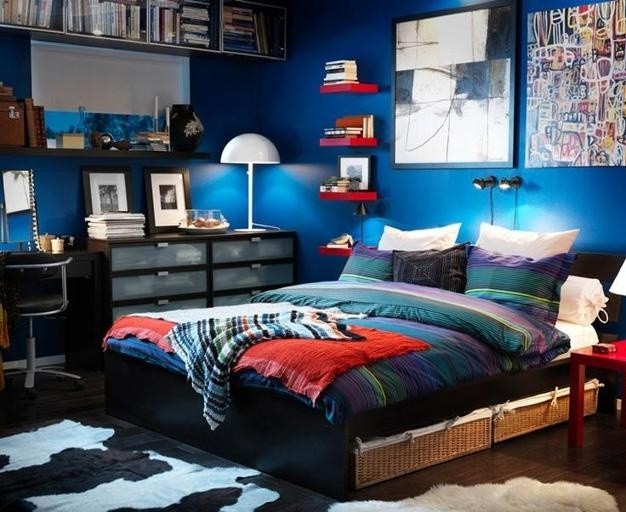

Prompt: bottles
[[50, 237, 64, 255], [73, 105, 92, 150]]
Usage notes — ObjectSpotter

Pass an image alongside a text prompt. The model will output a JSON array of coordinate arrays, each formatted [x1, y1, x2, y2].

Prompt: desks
[[566, 338, 625, 452], [0, 247, 102, 280]]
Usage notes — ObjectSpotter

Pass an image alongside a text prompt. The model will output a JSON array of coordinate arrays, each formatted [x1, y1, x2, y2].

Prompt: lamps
[[472, 175, 502, 225], [216, 130, 283, 236], [498, 175, 524, 231], [608, 258, 625, 299]]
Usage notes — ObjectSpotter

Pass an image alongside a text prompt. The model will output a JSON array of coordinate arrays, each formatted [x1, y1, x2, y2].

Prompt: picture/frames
[[81, 164, 134, 213], [392, 0, 520, 170], [336, 152, 373, 192], [144, 166, 193, 233]]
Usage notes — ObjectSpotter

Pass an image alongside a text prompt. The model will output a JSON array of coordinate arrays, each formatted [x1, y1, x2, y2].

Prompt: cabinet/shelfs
[[0, 0, 287, 60], [211, 235, 296, 311], [315, 78, 378, 261], [89, 239, 211, 330]]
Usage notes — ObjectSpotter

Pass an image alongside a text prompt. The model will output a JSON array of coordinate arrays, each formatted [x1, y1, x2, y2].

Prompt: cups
[[39, 234, 56, 254]]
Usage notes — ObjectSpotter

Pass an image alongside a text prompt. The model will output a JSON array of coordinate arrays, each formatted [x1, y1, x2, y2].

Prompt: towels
[[162, 308, 366, 433]]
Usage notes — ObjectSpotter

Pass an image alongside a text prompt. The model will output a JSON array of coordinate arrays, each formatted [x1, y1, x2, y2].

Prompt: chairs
[[0, 250, 83, 393]]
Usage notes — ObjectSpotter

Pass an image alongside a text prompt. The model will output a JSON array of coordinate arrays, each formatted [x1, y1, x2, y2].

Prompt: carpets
[[327, 474, 621, 512], [1, 409, 334, 512]]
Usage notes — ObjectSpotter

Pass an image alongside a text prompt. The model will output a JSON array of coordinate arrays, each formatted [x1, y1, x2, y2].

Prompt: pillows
[[464, 242, 574, 328], [387, 242, 472, 296], [557, 273, 612, 327], [378, 222, 462, 256], [338, 240, 396, 285], [475, 222, 580, 263]]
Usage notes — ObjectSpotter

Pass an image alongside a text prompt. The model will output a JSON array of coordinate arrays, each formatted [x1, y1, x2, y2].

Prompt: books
[[323, 56, 362, 85], [329, 232, 354, 246], [84, 207, 148, 243], [319, 184, 347, 193], [132, 127, 170, 152], [321, 175, 360, 186], [322, 111, 375, 138], [32, 106, 41, 148], [326, 242, 349, 249], [38, 106, 47, 148], [0, 0, 289, 58], [25, 97, 37, 148]]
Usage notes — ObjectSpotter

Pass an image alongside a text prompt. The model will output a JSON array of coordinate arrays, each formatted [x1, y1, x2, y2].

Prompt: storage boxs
[[0, 100, 27, 148]]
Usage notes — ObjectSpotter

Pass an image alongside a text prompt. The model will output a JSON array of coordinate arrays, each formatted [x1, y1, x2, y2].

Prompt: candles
[[165, 106, 170, 128], [154, 96, 159, 120]]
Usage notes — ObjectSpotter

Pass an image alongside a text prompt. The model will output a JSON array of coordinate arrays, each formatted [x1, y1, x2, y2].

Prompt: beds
[[100, 233, 605, 488]]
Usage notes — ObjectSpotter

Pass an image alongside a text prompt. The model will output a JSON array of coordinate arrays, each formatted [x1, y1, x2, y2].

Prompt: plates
[[178, 226, 229, 235]]
